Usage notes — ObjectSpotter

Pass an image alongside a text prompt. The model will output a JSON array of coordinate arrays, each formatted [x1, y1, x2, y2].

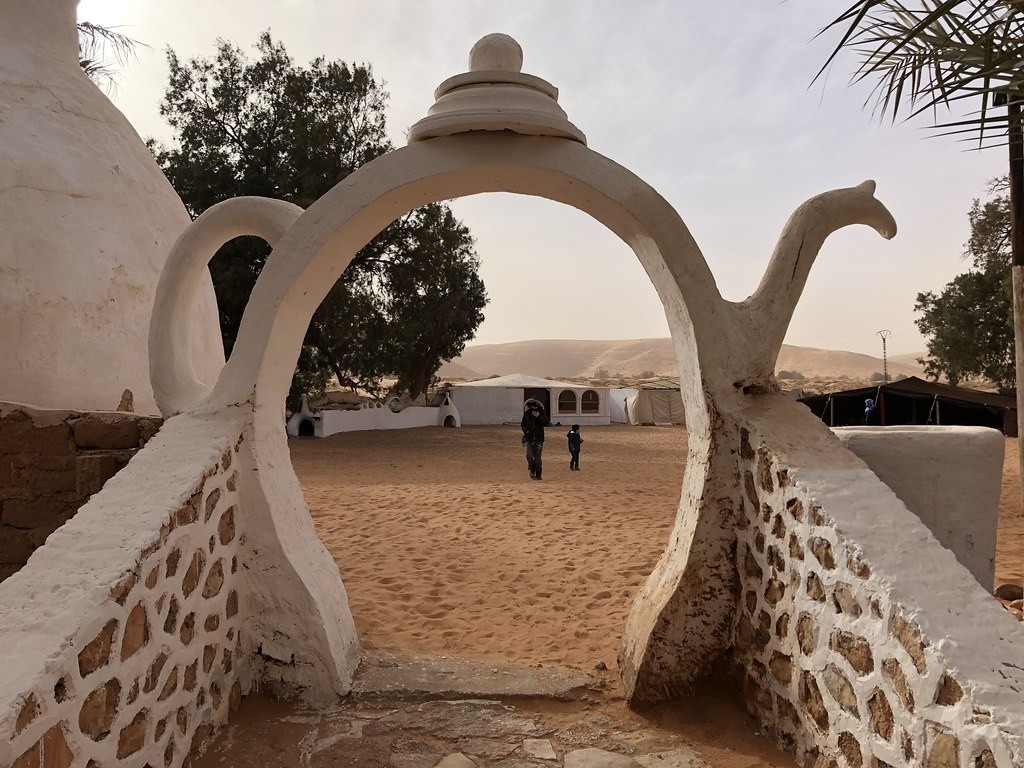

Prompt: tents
[[609, 376, 686, 426], [790, 375, 1018, 442]]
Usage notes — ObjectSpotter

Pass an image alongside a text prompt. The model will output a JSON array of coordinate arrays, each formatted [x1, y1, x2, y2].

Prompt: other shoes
[[535, 474, 542, 480], [571, 467, 580, 471], [528, 470, 536, 479]]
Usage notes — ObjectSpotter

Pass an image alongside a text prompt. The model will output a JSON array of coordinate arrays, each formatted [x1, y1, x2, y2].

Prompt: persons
[[567, 424, 583, 471], [520, 397, 549, 480], [863, 398, 880, 426]]
